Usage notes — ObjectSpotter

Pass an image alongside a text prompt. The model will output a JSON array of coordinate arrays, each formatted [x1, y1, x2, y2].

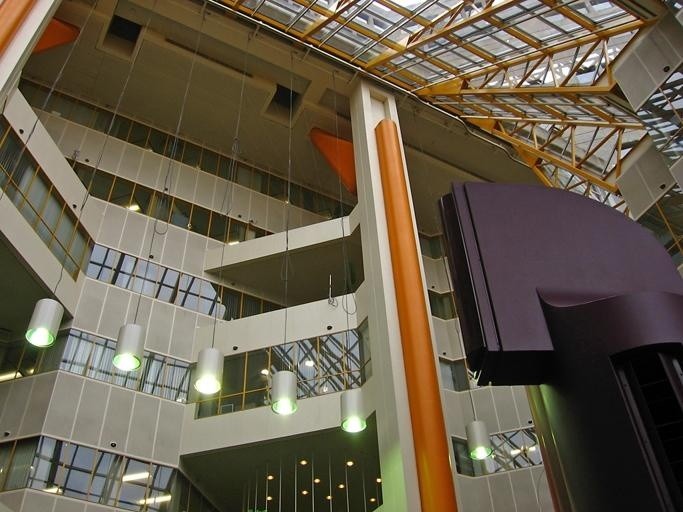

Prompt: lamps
[[331, 68, 368, 434], [271, 48, 297, 416], [193, 31, 252, 396], [111, 11, 209, 374], [25, 14, 155, 349], [431, 198, 492, 461]]
